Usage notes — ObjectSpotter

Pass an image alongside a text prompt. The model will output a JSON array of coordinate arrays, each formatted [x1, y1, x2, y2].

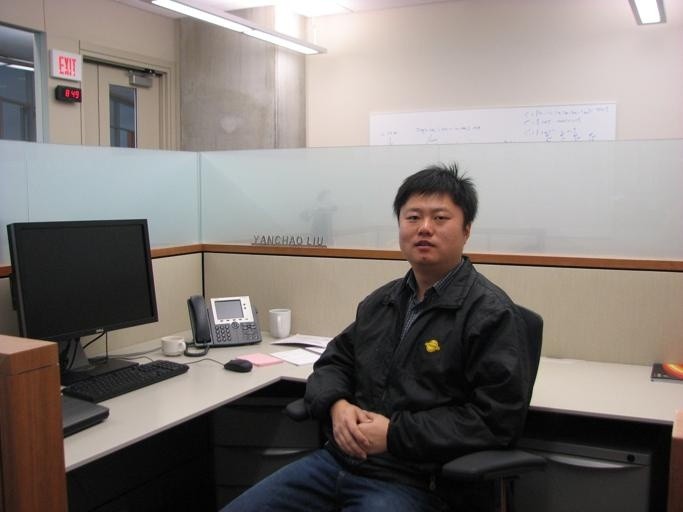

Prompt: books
[[650, 362, 683, 384]]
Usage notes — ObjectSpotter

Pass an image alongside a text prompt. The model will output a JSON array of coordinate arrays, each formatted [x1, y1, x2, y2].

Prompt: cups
[[268, 309, 292, 338], [161, 336, 186, 357]]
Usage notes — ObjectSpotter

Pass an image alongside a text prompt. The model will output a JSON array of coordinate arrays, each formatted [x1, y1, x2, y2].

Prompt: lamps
[[145, 1, 326, 57]]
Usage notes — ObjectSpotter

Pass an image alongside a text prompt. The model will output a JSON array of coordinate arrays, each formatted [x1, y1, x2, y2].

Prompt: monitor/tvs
[[6, 219, 158, 387]]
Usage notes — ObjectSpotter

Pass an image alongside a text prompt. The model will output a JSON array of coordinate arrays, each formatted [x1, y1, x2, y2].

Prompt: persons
[[217, 163, 531, 512]]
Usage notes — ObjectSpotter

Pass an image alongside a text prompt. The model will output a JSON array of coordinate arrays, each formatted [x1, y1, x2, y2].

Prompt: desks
[[61, 330, 683, 511]]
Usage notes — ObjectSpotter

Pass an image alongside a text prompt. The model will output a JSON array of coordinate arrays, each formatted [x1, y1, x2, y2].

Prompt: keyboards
[[61, 360, 189, 405]]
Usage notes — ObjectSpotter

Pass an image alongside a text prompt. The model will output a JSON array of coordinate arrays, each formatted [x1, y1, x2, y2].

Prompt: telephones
[[187, 295, 262, 347]]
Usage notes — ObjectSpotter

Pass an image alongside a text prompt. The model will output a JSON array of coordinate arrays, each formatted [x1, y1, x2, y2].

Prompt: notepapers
[[236, 353, 283, 367]]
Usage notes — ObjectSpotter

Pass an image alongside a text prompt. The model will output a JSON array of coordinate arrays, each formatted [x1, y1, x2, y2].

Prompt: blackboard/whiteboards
[[368, 102, 616, 145]]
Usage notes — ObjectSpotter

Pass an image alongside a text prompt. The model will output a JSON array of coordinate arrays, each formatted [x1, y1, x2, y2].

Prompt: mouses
[[225, 359, 252, 373]]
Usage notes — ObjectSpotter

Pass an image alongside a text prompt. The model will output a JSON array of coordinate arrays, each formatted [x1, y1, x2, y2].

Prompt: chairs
[[259, 305, 544, 510]]
